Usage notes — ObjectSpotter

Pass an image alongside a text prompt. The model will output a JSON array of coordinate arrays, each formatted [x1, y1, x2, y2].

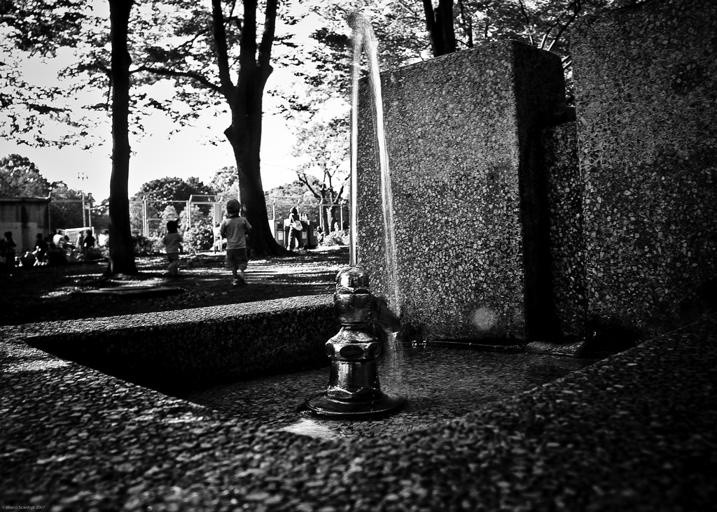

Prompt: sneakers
[[230, 278, 248, 287]]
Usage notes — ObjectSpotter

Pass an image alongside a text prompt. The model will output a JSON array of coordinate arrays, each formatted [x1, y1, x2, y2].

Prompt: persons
[[1, 226, 109, 286], [285, 207, 304, 252], [161, 219, 185, 277], [219, 199, 252, 286]]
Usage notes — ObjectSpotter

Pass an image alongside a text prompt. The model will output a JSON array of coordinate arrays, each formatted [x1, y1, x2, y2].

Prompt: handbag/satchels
[[292, 220, 303, 231]]
